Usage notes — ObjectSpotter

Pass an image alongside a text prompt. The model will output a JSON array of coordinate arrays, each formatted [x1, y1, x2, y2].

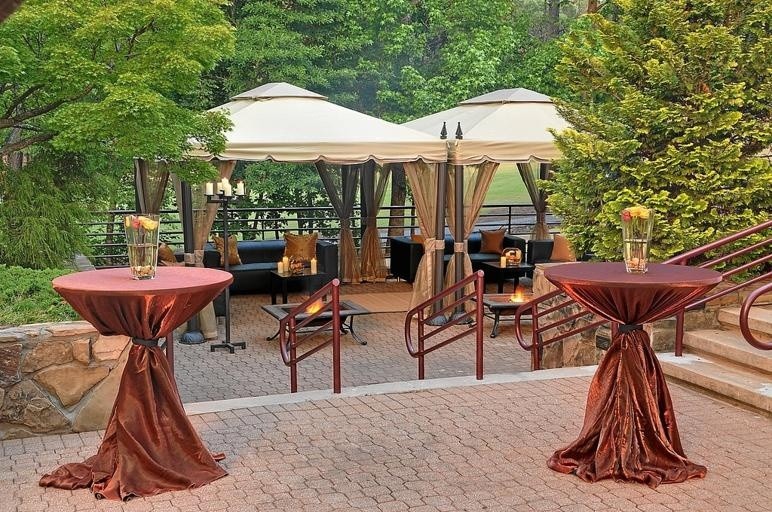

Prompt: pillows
[[410, 233, 426, 255], [478, 228, 507, 255], [550, 234, 577, 262], [280, 230, 319, 268], [158, 241, 178, 266], [211, 234, 243, 267]]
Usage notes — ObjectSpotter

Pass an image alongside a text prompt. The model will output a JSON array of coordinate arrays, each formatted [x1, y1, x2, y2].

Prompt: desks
[[51, 267, 234, 480], [544, 261, 724, 480]]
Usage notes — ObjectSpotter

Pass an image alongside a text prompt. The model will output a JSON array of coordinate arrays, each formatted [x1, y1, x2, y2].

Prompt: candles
[[282, 256, 289, 272], [277, 263, 284, 274], [500, 256, 507, 268], [205, 177, 245, 197], [310, 259, 318, 275]]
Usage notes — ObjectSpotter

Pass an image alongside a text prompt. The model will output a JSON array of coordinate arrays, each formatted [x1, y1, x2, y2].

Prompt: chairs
[[526, 239, 595, 279]]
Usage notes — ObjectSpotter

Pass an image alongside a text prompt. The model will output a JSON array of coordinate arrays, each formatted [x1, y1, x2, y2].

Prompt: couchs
[[155, 239, 339, 296], [388, 235, 526, 284]]
